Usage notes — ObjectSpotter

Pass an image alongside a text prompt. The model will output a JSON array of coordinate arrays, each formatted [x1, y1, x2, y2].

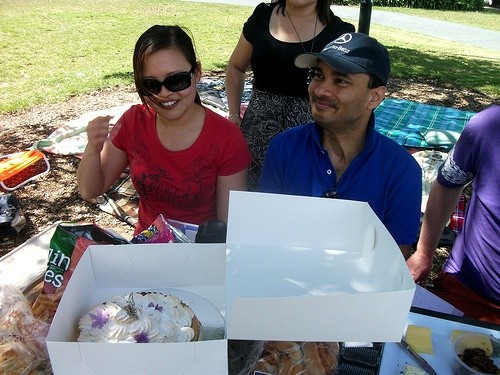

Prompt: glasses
[[141, 65, 194, 97]]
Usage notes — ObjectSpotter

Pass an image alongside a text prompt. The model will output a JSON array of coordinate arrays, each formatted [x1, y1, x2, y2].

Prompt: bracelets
[[227, 113, 242, 120]]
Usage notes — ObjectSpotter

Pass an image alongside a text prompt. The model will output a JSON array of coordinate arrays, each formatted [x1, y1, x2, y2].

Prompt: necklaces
[[285, 8, 319, 84]]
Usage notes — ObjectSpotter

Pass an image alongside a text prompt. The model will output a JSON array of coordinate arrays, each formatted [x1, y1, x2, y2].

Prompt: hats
[[294, 33, 390, 85]]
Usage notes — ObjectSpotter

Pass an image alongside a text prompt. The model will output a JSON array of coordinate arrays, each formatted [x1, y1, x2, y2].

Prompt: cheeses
[[451, 329, 492, 356], [405, 325, 433, 354]]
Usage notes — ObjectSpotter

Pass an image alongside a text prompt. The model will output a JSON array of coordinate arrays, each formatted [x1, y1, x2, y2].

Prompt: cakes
[[76, 292, 203, 343]]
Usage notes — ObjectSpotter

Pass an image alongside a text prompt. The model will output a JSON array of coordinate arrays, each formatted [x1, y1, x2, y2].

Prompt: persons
[[226, 0, 357, 192], [255, 31, 424, 260], [406, 106, 500, 308], [77, 25, 253, 236]]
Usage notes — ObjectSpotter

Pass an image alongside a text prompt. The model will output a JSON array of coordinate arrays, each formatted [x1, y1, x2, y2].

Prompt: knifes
[[401, 339, 437, 375]]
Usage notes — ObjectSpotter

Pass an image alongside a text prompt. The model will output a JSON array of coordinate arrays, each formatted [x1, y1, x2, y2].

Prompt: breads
[[456, 348, 500, 375], [251, 341, 339, 375], [0, 308, 51, 375]]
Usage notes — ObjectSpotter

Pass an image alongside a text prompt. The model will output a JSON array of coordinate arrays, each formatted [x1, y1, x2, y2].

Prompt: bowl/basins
[[452, 332, 500, 375]]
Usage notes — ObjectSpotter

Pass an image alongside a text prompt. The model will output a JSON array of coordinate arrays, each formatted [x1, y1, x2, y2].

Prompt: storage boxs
[[45, 190, 415, 375]]
